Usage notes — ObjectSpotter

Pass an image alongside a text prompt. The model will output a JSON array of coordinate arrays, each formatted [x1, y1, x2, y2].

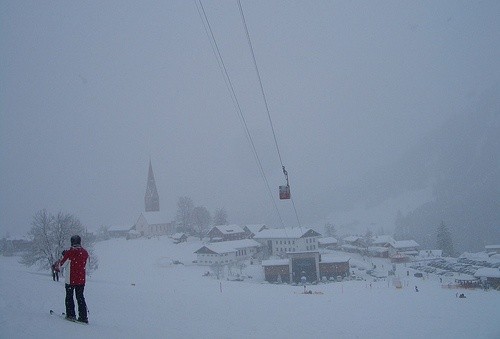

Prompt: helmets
[[70, 235, 81, 246]]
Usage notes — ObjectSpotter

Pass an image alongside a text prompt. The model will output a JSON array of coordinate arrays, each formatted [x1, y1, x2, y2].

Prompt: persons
[[60, 235, 89, 323], [51, 262, 60, 282]]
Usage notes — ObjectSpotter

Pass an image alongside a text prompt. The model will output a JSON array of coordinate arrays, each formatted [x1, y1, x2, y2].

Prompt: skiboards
[[49, 309, 89, 325]]
